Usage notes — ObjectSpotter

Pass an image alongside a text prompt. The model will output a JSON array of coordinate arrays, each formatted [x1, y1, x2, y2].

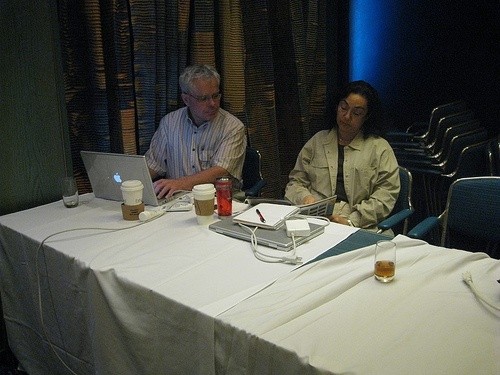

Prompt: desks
[[0, 191, 500, 375]]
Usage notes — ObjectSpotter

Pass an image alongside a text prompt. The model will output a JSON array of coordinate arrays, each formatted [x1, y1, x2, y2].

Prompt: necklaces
[[337, 133, 355, 144]]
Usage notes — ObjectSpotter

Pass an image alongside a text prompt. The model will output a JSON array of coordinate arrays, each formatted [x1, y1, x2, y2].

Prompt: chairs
[[242, 148, 268, 202], [377, 97, 500, 259]]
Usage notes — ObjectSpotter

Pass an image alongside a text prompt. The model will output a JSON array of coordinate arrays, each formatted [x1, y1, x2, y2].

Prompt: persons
[[283, 80, 402, 238], [130, 63, 248, 201]]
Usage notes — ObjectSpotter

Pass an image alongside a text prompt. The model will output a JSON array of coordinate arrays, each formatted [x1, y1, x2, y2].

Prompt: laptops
[[79, 151, 189, 206], [209, 207, 326, 251]]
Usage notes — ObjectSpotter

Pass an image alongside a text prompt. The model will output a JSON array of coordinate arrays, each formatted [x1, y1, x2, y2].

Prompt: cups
[[374, 240, 396, 282], [191, 183, 216, 225], [216, 176, 233, 217], [61, 176, 78, 207], [119, 180, 144, 206]]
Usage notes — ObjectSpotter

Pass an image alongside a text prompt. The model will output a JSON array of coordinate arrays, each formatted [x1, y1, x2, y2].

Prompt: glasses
[[184, 92, 222, 103]]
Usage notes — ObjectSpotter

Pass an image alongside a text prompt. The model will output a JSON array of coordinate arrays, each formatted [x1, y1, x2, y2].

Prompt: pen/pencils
[[256, 209, 266, 223]]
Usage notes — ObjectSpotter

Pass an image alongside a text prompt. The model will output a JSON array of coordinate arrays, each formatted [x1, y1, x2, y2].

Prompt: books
[[232, 202, 301, 231]]
